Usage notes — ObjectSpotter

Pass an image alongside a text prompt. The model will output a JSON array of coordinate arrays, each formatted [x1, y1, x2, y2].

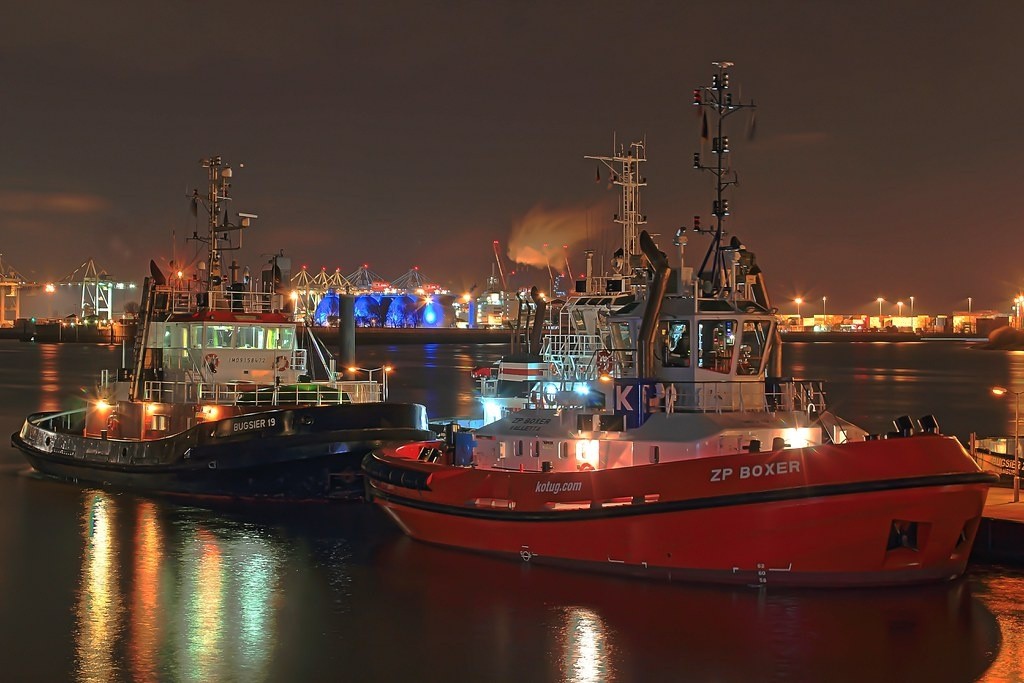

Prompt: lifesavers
[[596, 351, 612, 374], [544, 382, 559, 405], [204, 352, 220, 369], [272, 355, 289, 371]]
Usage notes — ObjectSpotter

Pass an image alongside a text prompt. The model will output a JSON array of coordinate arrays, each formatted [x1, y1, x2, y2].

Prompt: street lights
[[897, 301, 903, 317], [967, 296, 972, 322], [991, 387, 1024, 503], [877, 298, 885, 316], [909, 296, 915, 327], [822, 296, 827, 325], [796, 297, 801, 315]]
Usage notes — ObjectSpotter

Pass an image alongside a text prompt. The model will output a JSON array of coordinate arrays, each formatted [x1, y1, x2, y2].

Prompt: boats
[[8, 153, 439, 521], [967, 435, 1024, 483], [360, 59, 1001, 589]]
